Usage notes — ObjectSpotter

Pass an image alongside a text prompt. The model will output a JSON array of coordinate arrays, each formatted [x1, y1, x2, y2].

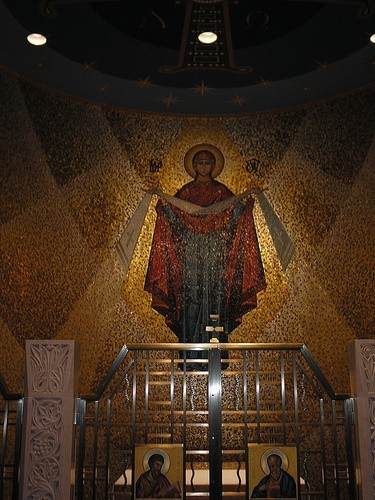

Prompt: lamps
[[370, 30, 375, 44], [26, 23, 48, 46], [197, 22, 219, 45]]
